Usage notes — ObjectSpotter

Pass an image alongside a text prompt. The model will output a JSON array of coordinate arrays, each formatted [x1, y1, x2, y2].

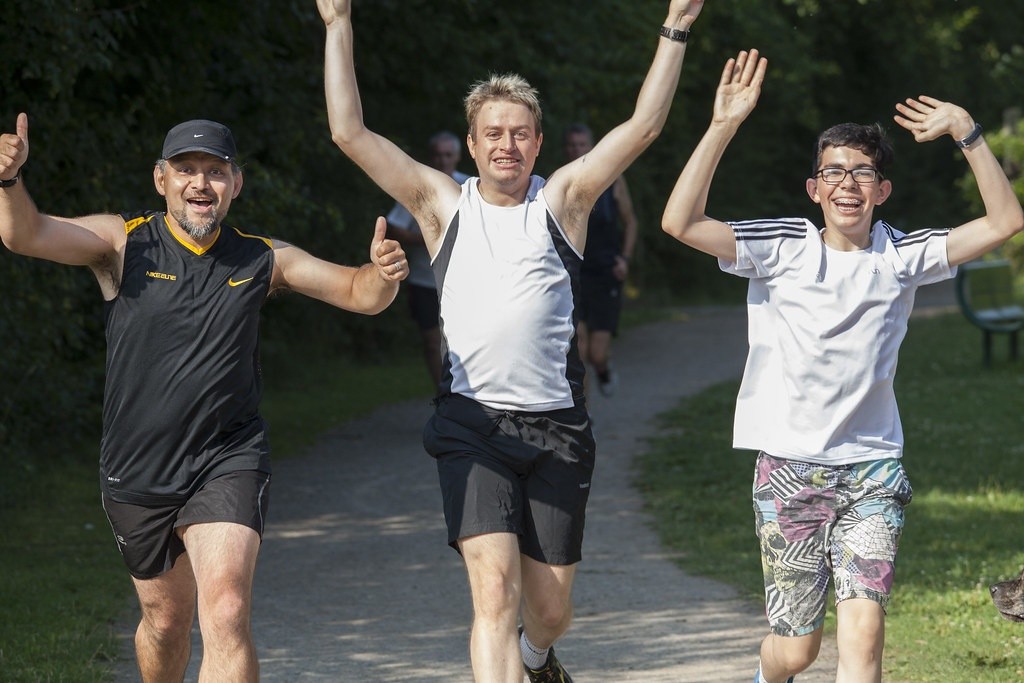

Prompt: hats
[[162, 120, 237, 163]]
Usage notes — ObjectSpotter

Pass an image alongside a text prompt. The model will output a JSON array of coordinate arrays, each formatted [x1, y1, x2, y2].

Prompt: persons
[[661, 46, 1024, 683], [380, 131, 471, 407], [315, 0, 705, 683], [0, 111, 409, 683], [557, 123, 640, 399]]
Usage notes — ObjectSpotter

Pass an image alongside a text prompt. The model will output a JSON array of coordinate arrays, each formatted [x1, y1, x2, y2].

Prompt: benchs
[[956, 258, 1024, 368]]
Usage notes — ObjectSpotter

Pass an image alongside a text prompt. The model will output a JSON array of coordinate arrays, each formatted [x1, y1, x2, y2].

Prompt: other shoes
[[596, 370, 613, 396]]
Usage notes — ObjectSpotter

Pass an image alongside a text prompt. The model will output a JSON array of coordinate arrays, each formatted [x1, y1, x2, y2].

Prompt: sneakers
[[518, 626, 574, 683]]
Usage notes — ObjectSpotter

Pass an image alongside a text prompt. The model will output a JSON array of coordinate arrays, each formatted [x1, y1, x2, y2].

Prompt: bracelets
[[660, 24, 691, 44], [1, 169, 23, 187], [954, 123, 983, 150]]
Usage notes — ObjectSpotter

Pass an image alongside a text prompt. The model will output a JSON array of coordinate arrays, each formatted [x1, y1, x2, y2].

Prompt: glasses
[[814, 168, 884, 183]]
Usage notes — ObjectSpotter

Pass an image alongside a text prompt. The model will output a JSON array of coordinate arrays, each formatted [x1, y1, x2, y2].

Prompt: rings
[[396, 262, 402, 270]]
